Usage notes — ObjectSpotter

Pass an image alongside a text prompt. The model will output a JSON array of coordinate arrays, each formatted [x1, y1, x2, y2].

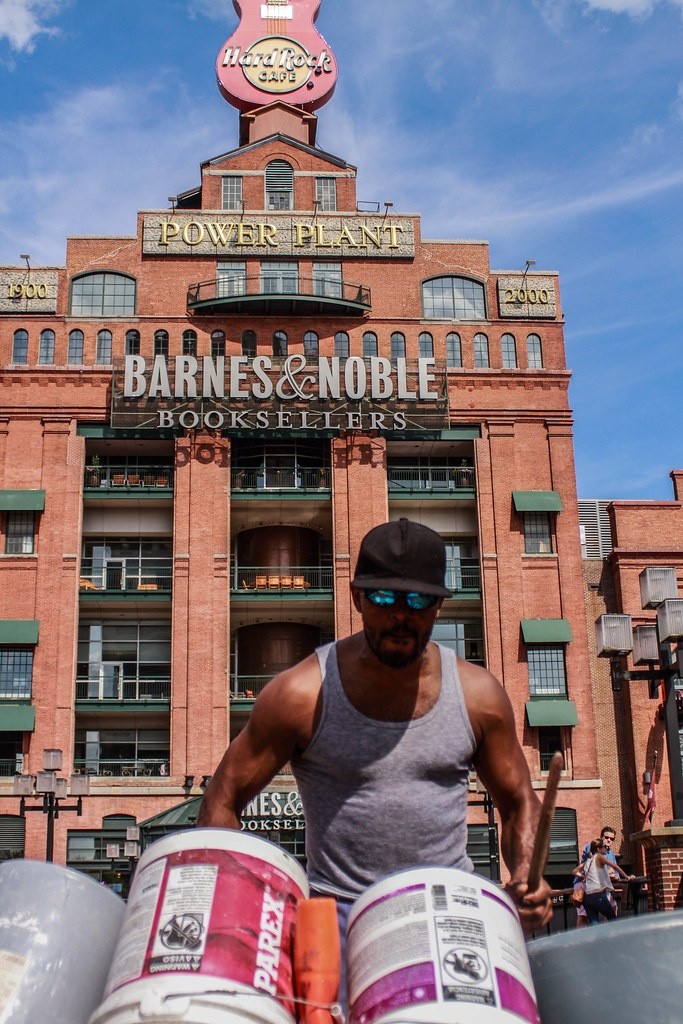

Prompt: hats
[[351, 517, 452, 598]]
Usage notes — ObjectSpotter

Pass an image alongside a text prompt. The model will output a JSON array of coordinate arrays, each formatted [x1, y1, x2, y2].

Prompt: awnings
[[0, 705, 35, 731], [0, 489, 45, 510], [520, 618, 573, 645], [0, 619, 39, 643], [512, 490, 563, 512], [525, 701, 580, 726]]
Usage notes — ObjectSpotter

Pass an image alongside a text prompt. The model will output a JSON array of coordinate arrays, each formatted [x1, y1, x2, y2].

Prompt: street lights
[[594, 565, 683, 826], [12, 748, 90, 863]]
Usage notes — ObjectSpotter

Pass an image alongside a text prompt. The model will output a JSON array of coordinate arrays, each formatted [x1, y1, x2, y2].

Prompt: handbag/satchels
[[571, 878, 586, 907]]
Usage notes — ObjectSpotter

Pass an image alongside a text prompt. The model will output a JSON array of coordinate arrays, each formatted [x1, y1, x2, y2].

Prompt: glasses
[[601, 845, 607, 849], [361, 588, 439, 610], [604, 836, 614, 841]]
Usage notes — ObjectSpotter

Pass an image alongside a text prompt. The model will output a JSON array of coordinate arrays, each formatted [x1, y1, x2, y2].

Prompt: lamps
[[312, 200, 322, 211], [202, 775, 212, 787], [167, 197, 177, 209], [523, 260, 536, 278], [184, 775, 195, 787], [240, 199, 248, 210], [384, 202, 393, 214], [20, 255, 31, 267]]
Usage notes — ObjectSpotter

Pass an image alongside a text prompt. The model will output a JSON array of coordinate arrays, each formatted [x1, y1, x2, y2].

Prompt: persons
[[195, 517, 554, 934], [573, 827, 648, 929]]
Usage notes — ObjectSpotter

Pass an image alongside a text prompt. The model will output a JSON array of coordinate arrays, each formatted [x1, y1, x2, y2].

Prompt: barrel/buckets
[[88, 827, 344, 1024], [0, 859, 126, 1024], [346, 866, 540, 1024]]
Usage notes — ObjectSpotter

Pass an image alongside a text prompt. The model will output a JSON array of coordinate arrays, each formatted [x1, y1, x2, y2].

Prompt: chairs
[[241, 580, 252, 589], [280, 576, 293, 590], [267, 576, 280, 589], [292, 576, 308, 590], [80, 578, 95, 590], [141, 476, 157, 488], [110, 475, 126, 487], [255, 575, 267, 589], [126, 475, 141, 488], [156, 476, 169, 488]]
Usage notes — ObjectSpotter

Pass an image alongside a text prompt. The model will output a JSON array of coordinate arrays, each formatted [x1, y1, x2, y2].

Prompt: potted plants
[[88, 454, 103, 487], [456, 459, 471, 488]]
